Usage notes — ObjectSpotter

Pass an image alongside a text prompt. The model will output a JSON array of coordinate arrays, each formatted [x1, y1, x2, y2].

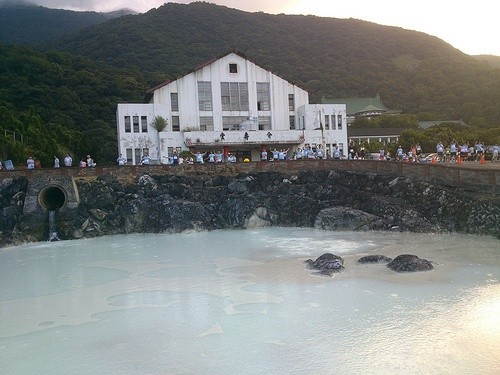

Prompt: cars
[[422, 153, 446, 162]]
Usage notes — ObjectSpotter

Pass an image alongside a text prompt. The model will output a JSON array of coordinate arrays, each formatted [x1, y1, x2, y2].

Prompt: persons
[[27, 156, 35, 170], [213, 151, 224, 162], [190, 151, 207, 163], [34, 158, 41, 168], [63, 153, 72, 167], [262, 148, 267, 161], [163, 154, 174, 164], [116, 153, 127, 165], [204, 150, 216, 163], [53, 155, 60, 168], [142, 153, 151, 165], [86, 155, 94, 167], [228, 154, 236, 162], [79, 158, 86, 167], [269, 141, 500, 163]]
